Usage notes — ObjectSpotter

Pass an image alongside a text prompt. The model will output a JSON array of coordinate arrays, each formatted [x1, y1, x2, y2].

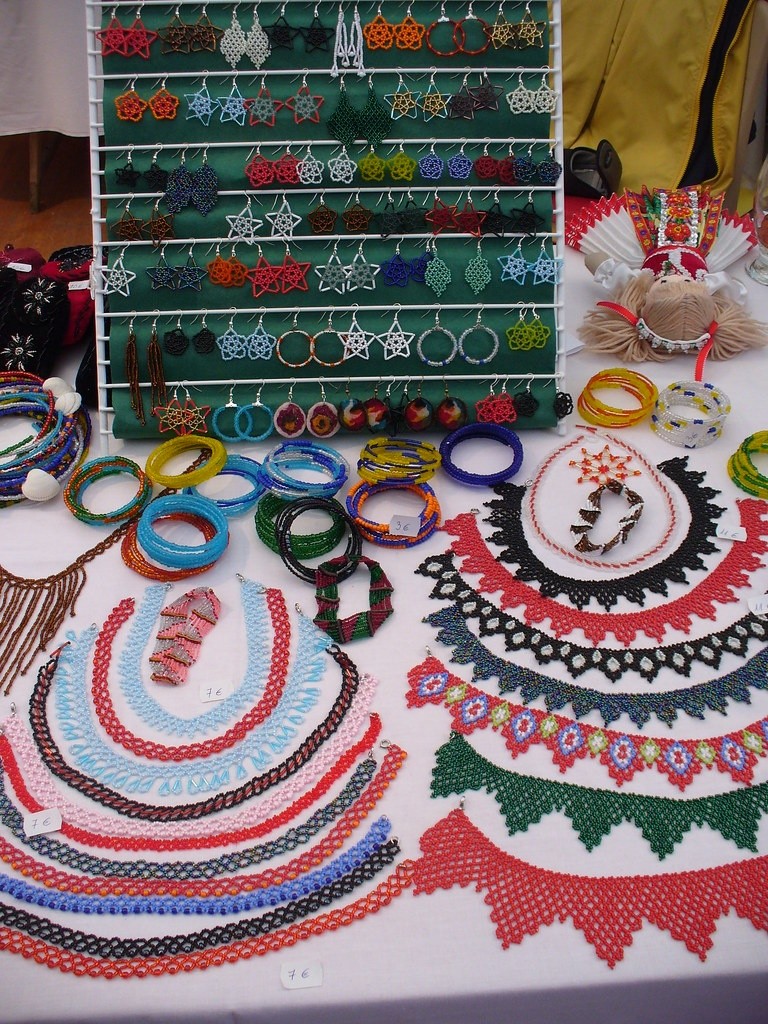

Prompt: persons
[[558, 182, 768, 364], [546, 0, 768, 219]]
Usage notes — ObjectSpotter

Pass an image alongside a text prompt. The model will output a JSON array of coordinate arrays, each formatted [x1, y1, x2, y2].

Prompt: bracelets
[[145, 434, 228, 489], [577, 366, 659, 429], [727, 430, 768, 500], [256, 491, 364, 585], [569, 480, 647, 555], [314, 555, 394, 645], [439, 422, 523, 484], [344, 436, 443, 484], [148, 584, 219, 685], [66, 453, 152, 528], [346, 476, 442, 550], [181, 452, 267, 516], [649, 379, 731, 449], [120, 492, 230, 583], [252, 441, 362, 502]]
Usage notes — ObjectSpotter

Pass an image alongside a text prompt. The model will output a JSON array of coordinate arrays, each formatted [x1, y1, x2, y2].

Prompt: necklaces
[[0, 573, 416, 977], [408, 456, 768, 969]]
